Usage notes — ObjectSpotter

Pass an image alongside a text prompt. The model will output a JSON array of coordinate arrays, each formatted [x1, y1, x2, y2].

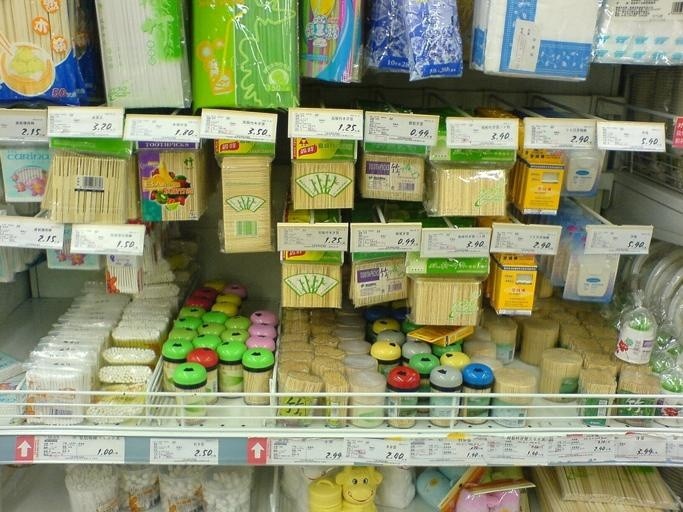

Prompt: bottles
[[163, 281, 278, 425], [368, 307, 495, 427]]
